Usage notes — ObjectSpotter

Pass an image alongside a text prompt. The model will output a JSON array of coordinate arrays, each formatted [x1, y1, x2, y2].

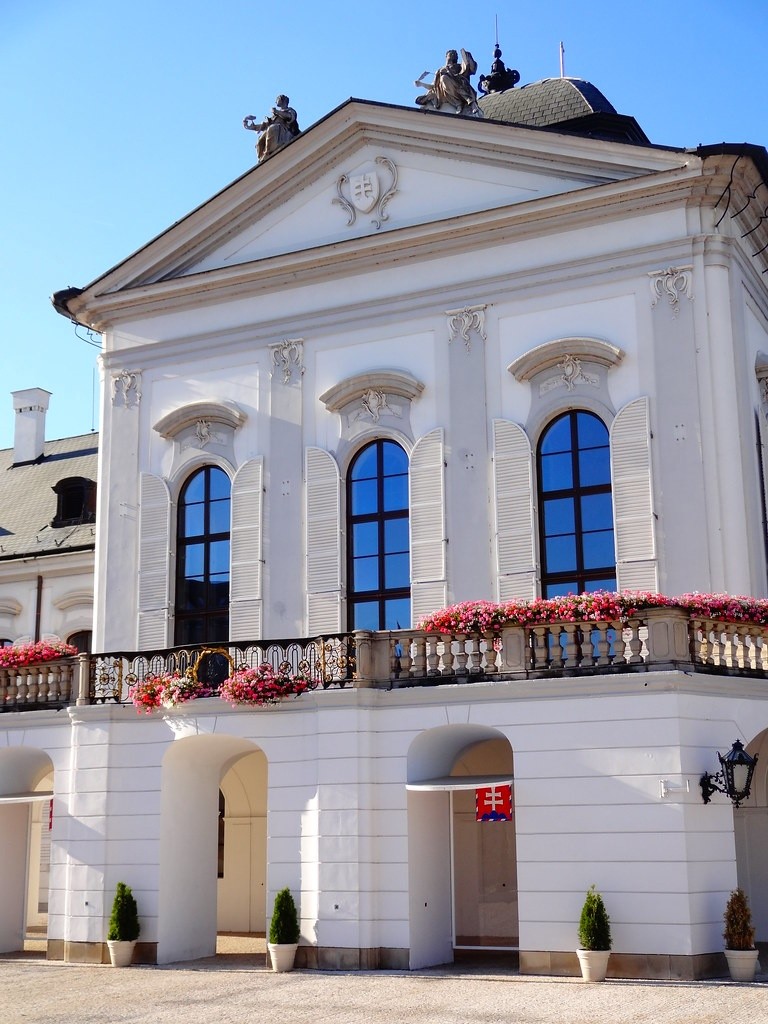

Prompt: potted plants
[[267, 886, 301, 970], [575, 883, 613, 983], [721, 884, 759, 982], [106, 882, 141, 967]]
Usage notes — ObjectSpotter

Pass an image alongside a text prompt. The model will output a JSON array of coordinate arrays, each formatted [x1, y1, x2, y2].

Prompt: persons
[[414, 47, 480, 115], [243, 94, 302, 164]]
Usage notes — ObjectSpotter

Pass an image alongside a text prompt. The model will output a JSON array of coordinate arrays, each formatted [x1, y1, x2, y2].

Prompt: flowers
[[215, 664, 320, 709], [0, 639, 79, 669], [128, 670, 210, 715], [415, 587, 768, 651]]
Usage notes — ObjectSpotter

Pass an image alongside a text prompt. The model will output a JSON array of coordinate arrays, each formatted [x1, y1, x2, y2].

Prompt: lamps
[[698, 738, 759, 810]]
